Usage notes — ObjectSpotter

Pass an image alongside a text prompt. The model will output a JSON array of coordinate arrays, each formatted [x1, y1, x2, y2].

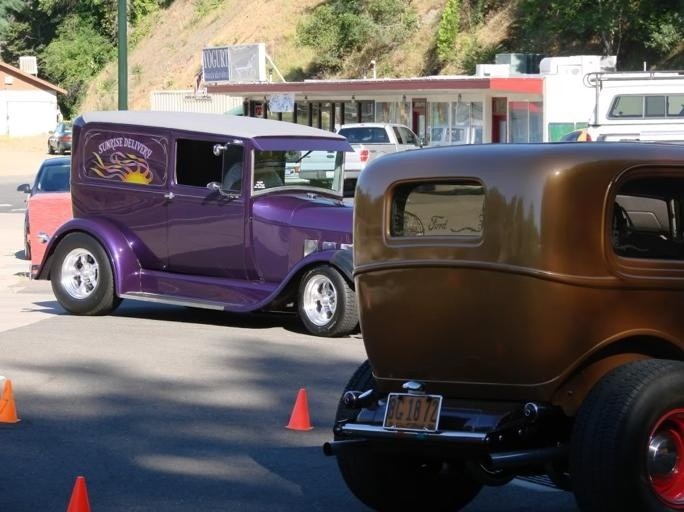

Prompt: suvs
[[34, 110, 360, 338], [320, 140, 683, 511]]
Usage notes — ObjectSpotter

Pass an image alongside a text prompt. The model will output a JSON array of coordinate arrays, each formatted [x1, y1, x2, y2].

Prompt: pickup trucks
[[297, 122, 428, 193]]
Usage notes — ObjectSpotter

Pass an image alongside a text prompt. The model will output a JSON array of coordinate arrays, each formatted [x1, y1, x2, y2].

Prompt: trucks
[[582, 70, 683, 144]]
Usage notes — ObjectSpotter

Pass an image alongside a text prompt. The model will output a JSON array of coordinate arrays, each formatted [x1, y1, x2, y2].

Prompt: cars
[[556, 127, 590, 144], [422, 125, 482, 148], [15, 155, 71, 259], [283, 151, 320, 187], [47, 120, 72, 156]]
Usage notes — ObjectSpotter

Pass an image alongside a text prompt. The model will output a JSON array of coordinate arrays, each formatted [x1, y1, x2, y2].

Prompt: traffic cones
[[284, 387, 313, 431], [0, 379, 21, 425], [65, 476, 91, 512]]
